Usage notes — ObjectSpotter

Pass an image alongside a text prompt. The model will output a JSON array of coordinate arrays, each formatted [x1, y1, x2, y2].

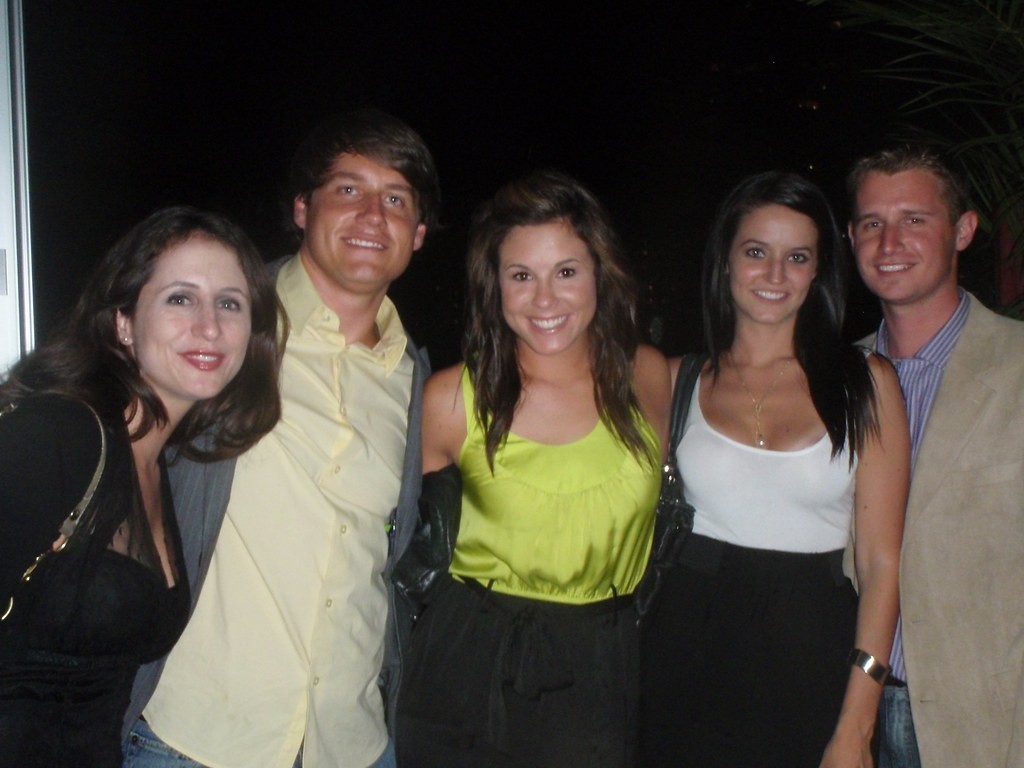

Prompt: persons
[[846, 142, 1023, 768], [0, 204, 291, 768], [122, 117, 433, 768], [669, 169, 912, 768], [396, 165, 672, 768]]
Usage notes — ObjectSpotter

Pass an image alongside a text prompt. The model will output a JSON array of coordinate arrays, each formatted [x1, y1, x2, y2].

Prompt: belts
[[883, 674, 906, 688]]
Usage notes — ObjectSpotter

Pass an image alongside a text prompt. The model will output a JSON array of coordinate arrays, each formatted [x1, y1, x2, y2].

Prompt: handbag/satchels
[[630, 352, 711, 634]]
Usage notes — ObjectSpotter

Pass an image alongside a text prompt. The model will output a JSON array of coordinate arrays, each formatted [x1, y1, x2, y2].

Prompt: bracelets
[[850, 648, 889, 687]]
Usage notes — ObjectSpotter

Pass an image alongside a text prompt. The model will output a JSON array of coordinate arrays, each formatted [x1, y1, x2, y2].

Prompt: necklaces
[[725, 353, 793, 447]]
